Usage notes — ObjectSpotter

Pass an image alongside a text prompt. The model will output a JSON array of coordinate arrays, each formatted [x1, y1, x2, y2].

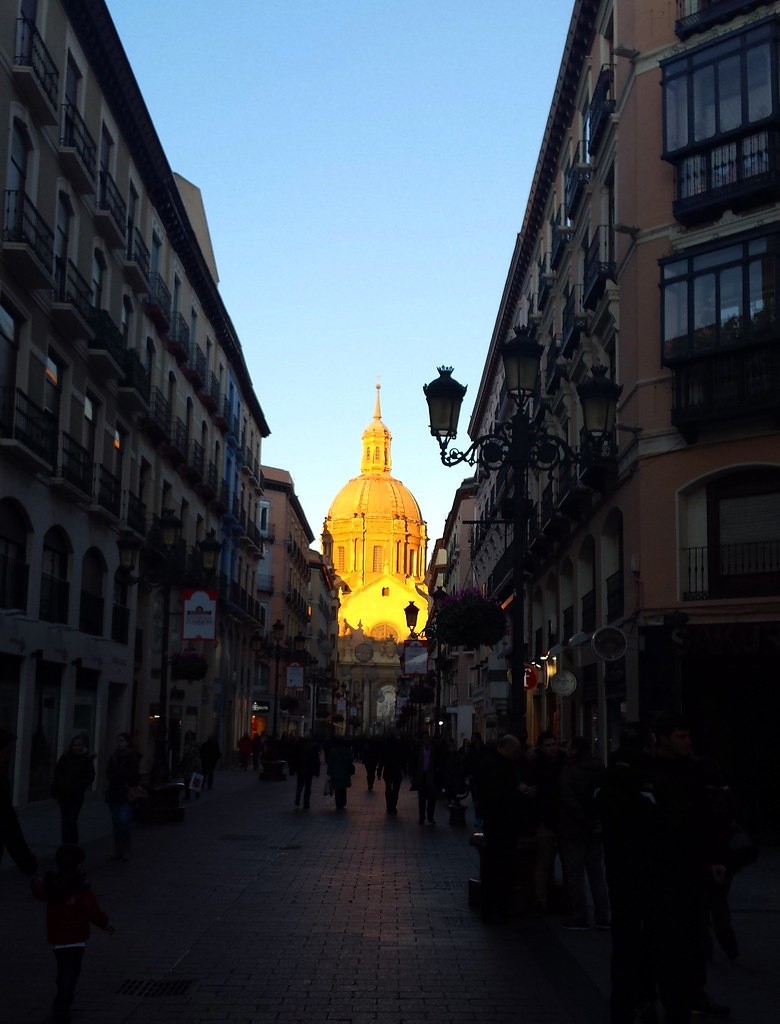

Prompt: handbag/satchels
[[323, 780, 333, 796], [347, 775, 351, 787], [726, 817, 757, 867], [188, 772, 204, 792]]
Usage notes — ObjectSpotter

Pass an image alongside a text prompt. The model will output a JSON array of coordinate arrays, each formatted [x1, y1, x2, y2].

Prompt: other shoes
[[109, 855, 131, 864], [54, 1014, 72, 1024], [591, 919, 612, 929], [418, 820, 425, 825], [530, 900, 547, 911], [640, 1003, 660, 1024], [724, 940, 740, 960], [691, 997, 731, 1018], [562, 919, 592, 930], [182, 796, 190, 800], [195, 793, 200, 799], [368, 786, 373, 792], [427, 816, 436, 824], [386, 805, 398, 817]]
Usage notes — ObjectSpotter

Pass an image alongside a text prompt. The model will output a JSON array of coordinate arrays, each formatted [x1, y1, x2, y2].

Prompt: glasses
[[117, 739, 124, 742]]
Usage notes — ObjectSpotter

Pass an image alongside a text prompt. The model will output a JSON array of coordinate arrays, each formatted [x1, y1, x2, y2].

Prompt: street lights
[[114, 506, 222, 781], [405, 584, 452, 733], [271, 619, 285, 761], [310, 656, 319, 733], [421, 323, 626, 747]]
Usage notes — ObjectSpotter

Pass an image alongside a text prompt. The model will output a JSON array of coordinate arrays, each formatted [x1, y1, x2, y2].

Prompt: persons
[[238, 731, 267, 772], [30, 845, 115, 1024], [289, 731, 483, 826], [0, 772, 44, 879], [476, 734, 610, 930], [200, 734, 221, 790], [603, 712, 740, 1024], [105, 732, 143, 862], [179, 730, 201, 801], [50, 733, 96, 850]]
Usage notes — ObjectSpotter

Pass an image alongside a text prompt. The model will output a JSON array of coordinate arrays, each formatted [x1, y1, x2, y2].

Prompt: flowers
[[171, 643, 210, 667], [438, 587, 508, 625]]
